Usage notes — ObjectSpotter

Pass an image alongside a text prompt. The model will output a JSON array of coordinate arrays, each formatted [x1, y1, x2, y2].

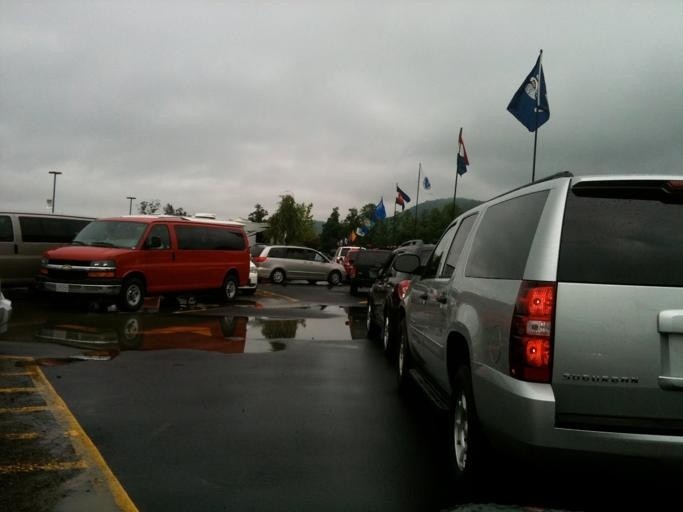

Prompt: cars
[[237, 252, 258, 293], [348, 250, 393, 296], [331, 247, 365, 265], [342, 304, 368, 340], [28, 308, 247, 368], [342, 250, 364, 284], [365, 239, 437, 359]]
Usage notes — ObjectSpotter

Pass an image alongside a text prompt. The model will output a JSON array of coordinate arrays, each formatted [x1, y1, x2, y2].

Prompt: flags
[[395, 185, 410, 212], [456, 135, 469, 176], [506, 52, 549, 132], [336, 197, 385, 247], [418, 163, 432, 194]]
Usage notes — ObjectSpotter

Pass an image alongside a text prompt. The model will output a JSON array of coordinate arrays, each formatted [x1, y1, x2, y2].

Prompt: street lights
[[46, 197, 51, 215], [47, 171, 62, 213], [127, 196, 136, 216]]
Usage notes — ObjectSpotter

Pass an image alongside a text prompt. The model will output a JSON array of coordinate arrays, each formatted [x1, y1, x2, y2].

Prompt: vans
[[249, 244, 347, 286], [0, 211, 95, 299], [393, 170, 682, 489], [37, 215, 249, 313]]
